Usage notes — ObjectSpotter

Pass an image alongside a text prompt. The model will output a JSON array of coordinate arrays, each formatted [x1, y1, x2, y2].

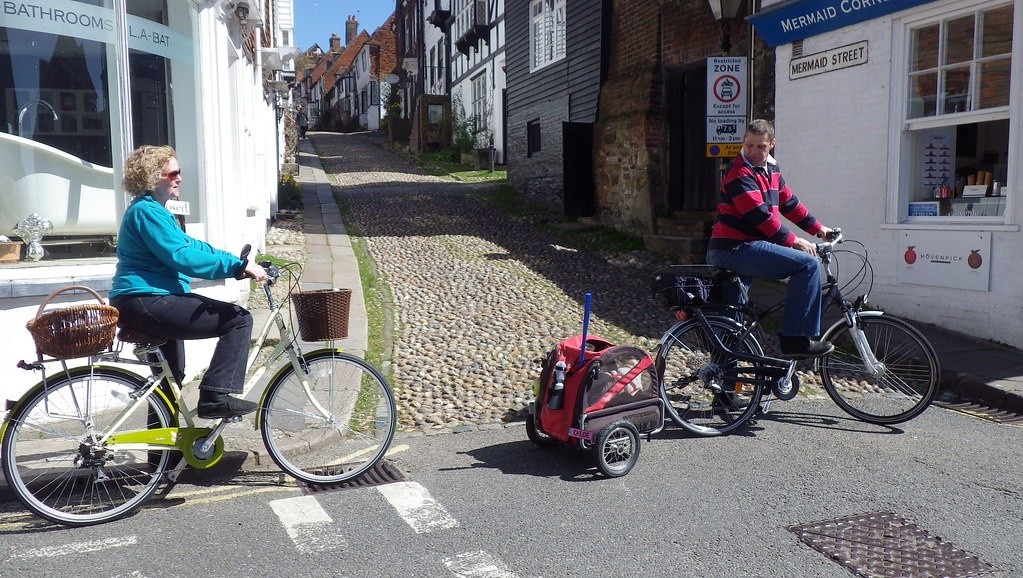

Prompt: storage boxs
[[0, 242, 22, 260]]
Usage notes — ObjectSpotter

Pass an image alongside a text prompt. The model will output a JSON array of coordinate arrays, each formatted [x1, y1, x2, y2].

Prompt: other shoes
[[304, 137, 309, 139], [148, 446, 206, 470], [299, 137, 303, 140], [197, 388, 259, 419]]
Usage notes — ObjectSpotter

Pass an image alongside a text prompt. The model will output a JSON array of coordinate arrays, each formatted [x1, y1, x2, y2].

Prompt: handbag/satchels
[[306, 121, 310, 124]]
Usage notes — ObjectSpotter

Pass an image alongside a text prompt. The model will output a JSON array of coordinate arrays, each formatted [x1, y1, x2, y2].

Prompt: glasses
[[161, 169, 181, 180]]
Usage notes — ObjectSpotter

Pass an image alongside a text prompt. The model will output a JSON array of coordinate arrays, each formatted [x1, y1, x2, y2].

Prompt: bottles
[[934, 184, 951, 199]]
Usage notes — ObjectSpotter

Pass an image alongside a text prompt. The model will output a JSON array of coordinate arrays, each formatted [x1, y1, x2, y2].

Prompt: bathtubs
[[0, 130, 116, 236]]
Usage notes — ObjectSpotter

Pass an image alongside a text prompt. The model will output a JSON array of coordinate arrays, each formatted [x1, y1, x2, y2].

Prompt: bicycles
[[0, 244, 399, 528], [655, 224, 941, 437]]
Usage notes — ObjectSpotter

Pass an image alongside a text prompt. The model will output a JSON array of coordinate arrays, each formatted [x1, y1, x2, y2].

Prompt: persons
[[297, 106, 310, 139], [706, 118, 836, 411], [107, 145, 268, 481]]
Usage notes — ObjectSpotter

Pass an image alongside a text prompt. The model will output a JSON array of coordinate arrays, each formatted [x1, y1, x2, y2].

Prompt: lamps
[[275, 105, 285, 124], [235, 3, 249, 20], [708, 0, 742, 52]]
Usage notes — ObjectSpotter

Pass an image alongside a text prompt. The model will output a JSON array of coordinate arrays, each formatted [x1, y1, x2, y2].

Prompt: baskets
[[26, 284, 119, 358], [291, 288, 352, 342], [650, 265, 725, 311]]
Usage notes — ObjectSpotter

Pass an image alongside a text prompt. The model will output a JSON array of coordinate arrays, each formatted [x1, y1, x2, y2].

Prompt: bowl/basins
[[967, 170, 993, 186]]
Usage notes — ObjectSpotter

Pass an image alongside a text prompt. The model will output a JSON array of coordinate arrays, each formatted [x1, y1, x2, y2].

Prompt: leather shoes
[[711, 392, 750, 411], [776, 331, 835, 358]]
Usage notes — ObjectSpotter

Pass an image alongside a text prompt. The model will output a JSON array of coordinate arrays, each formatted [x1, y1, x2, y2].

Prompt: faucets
[[17, 100, 59, 137]]
